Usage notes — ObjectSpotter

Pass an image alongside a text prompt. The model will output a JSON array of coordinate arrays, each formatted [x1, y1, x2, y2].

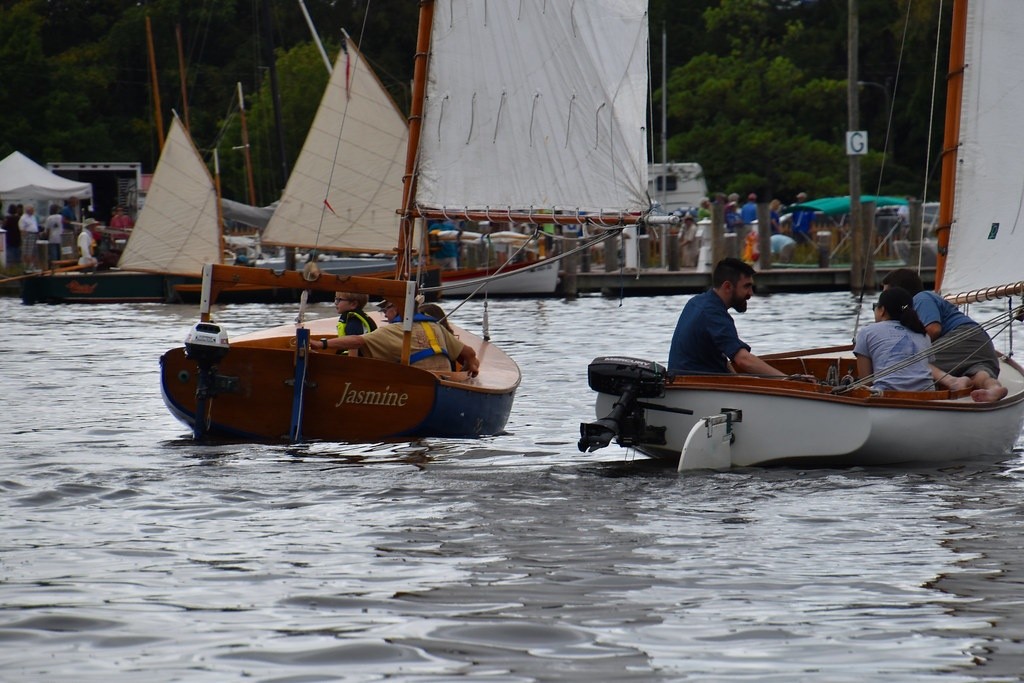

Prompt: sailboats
[[160, 0, 678, 441], [577, 1, 1024, 473], [19, 0, 560, 300]]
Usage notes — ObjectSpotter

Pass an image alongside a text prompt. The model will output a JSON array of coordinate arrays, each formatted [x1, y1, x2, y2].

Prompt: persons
[[511, 222, 530, 234], [679, 215, 697, 252], [769, 200, 785, 234], [60, 197, 78, 227], [853, 287, 937, 391], [668, 257, 784, 377], [428, 221, 463, 268], [881, 268, 1008, 402], [488, 221, 510, 232], [45, 205, 63, 268], [309, 299, 478, 378], [2, 204, 24, 263], [726, 193, 757, 234], [77, 218, 99, 273], [419, 304, 453, 334], [792, 192, 817, 241], [18, 204, 42, 275], [335, 292, 378, 357], [109, 207, 131, 247], [698, 197, 711, 219]]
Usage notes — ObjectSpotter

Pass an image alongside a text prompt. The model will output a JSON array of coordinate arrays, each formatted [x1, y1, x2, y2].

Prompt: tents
[[0, 151, 93, 210]]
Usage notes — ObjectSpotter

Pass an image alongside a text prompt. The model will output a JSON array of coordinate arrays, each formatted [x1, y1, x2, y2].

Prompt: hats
[[376, 299, 388, 307], [83, 218, 99, 227]]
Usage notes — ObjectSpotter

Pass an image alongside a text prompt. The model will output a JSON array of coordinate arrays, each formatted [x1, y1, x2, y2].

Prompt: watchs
[[320, 338, 327, 350]]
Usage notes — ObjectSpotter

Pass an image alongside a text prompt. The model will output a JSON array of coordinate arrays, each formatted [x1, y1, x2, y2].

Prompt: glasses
[[872, 303, 883, 311], [383, 304, 393, 313], [334, 296, 353, 302]]
